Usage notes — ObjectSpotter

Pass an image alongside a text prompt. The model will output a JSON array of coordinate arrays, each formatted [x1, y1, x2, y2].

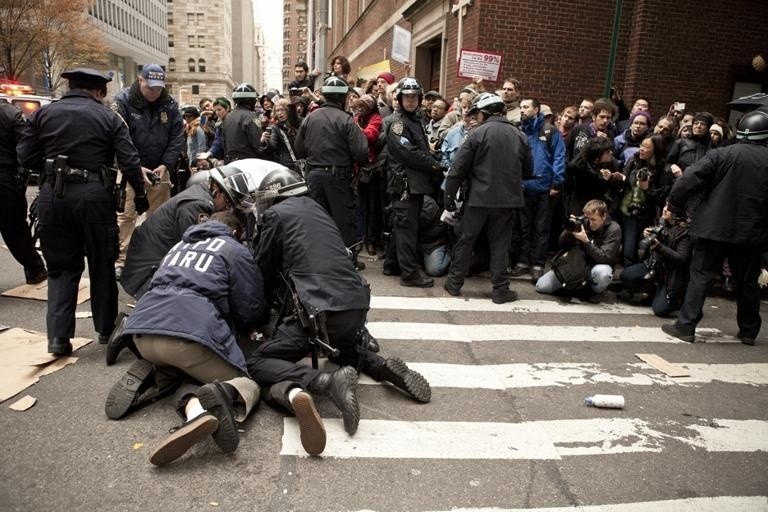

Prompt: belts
[[42, 170, 100, 183]]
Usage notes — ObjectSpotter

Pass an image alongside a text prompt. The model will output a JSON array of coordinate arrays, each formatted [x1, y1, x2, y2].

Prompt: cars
[[0, 83, 49, 116]]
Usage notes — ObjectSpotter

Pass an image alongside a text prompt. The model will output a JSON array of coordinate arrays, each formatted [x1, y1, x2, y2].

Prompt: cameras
[[291, 88, 303, 96], [266, 127, 272, 136], [626, 202, 645, 219], [200, 115, 206, 125], [640, 226, 673, 251], [148, 172, 161, 186], [638, 170, 649, 180], [567, 214, 590, 233]]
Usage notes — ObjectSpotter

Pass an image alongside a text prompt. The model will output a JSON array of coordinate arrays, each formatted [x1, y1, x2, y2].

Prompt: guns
[[270, 271, 340, 370]]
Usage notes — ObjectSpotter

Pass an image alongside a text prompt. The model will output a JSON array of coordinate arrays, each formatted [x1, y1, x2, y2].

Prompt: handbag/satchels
[[549, 244, 592, 287]]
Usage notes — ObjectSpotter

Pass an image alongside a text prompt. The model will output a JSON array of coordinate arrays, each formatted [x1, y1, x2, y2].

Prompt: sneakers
[[329, 365, 360, 436], [662, 321, 694, 342], [385, 356, 432, 403], [149, 411, 219, 465], [26, 267, 48, 284], [382, 266, 544, 304], [737, 330, 755, 345], [115, 265, 124, 281], [103, 360, 154, 420], [197, 381, 239, 454], [293, 392, 327, 455]]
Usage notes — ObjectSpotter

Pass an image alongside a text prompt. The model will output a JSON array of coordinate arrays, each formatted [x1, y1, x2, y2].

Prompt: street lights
[[178, 86, 187, 105]]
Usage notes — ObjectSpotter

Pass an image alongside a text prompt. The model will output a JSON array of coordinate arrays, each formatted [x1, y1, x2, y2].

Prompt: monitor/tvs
[[673, 102, 686, 111]]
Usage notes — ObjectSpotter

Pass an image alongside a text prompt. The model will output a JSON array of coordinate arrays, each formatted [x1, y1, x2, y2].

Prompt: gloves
[[134, 195, 150, 216], [444, 199, 458, 212], [437, 163, 449, 172], [671, 211, 689, 223]]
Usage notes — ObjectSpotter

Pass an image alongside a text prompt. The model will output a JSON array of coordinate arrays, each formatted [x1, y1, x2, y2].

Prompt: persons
[[17, 69, 150, 355], [0, 103, 47, 284]]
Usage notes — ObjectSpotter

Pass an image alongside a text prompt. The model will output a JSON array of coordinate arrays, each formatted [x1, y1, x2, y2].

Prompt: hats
[[377, 72, 394, 85], [142, 63, 166, 89], [352, 87, 377, 110], [182, 106, 200, 120], [540, 104, 553, 117], [60, 68, 112, 84], [425, 90, 441, 99]]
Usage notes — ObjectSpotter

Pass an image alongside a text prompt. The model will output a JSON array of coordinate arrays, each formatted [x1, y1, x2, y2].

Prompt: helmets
[[396, 76, 423, 98], [321, 76, 349, 93], [736, 110, 768, 142], [466, 93, 505, 118], [231, 84, 257, 101], [258, 168, 308, 213], [208, 166, 249, 205]]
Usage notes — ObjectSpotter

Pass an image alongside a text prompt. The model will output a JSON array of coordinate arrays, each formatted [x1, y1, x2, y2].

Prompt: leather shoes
[[48, 338, 73, 354], [357, 326, 379, 352], [98, 331, 110, 344]]
